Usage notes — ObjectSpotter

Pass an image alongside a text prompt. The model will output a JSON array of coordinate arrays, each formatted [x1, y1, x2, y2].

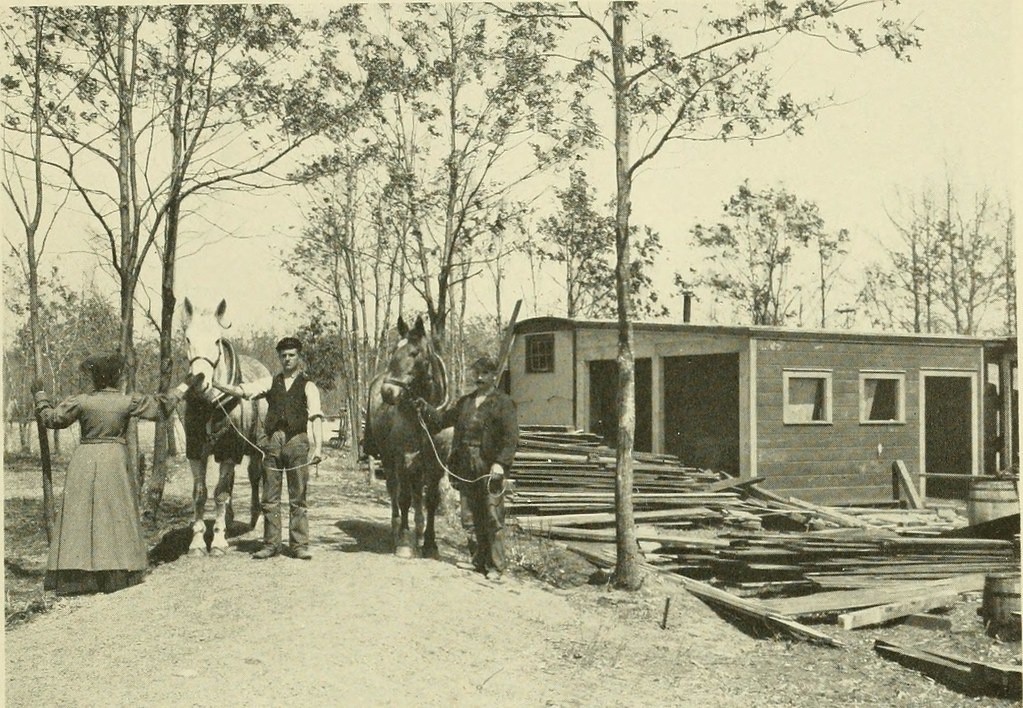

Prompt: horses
[[183, 298, 273, 559], [363, 317, 455, 560]]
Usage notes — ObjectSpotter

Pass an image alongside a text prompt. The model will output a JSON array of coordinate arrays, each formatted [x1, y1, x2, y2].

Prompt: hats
[[275, 338, 302, 352]]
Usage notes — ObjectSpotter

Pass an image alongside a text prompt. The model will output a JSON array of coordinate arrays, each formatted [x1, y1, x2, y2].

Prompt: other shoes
[[456, 561, 480, 571], [295, 549, 312, 559], [487, 569, 501, 580], [253, 547, 276, 559]]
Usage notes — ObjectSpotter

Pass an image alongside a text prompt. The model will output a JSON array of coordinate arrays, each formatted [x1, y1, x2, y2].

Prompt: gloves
[[185, 376, 197, 387], [30, 377, 45, 394]]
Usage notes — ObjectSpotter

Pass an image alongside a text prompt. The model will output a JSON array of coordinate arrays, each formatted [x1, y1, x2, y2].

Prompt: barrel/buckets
[[984, 570, 1022, 639], [969, 478, 1020, 538]]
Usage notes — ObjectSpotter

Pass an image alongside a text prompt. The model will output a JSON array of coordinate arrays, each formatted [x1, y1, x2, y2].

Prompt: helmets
[[469, 355, 500, 373]]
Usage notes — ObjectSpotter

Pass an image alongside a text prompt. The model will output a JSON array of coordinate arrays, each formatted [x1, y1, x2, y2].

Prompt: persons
[[409, 355, 519, 581], [31, 357, 205, 594], [212, 337, 324, 560]]
